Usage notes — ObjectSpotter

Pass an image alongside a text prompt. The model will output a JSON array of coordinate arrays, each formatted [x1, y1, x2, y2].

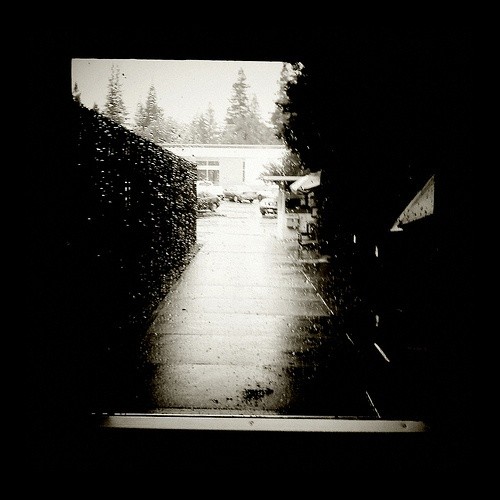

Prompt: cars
[[259, 196, 280, 216], [222, 184, 257, 204], [197, 180, 224, 200], [255, 186, 280, 201], [197, 190, 219, 212]]
